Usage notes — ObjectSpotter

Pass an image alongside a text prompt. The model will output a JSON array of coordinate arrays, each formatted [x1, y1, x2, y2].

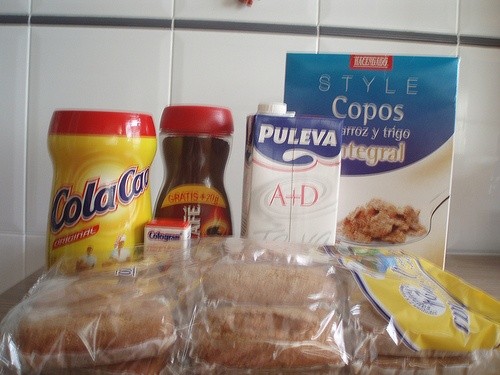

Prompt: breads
[[18, 258, 475, 375]]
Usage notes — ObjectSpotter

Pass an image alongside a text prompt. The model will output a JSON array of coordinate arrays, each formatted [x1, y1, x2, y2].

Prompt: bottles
[[47, 110, 158, 276], [152, 104, 234, 240], [239, 102, 344, 247]]
